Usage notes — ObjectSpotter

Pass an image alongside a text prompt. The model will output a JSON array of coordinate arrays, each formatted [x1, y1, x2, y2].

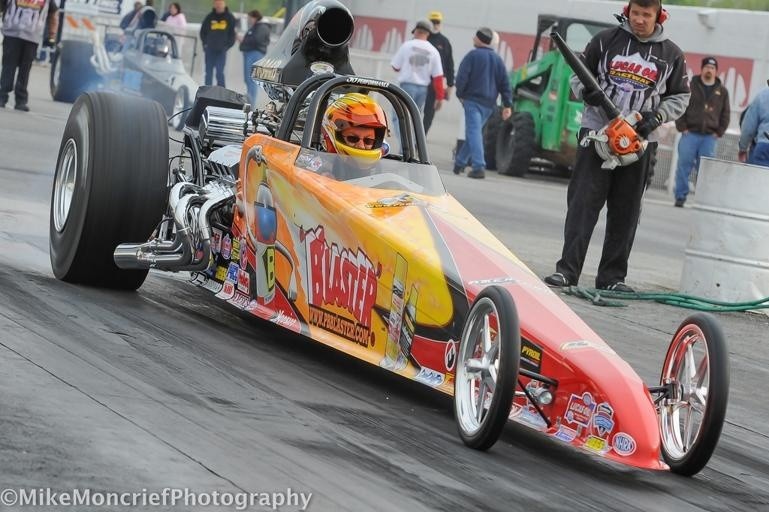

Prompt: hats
[[426, 9, 443, 22], [701, 55, 718, 71], [411, 20, 432, 35], [475, 26, 493, 44]]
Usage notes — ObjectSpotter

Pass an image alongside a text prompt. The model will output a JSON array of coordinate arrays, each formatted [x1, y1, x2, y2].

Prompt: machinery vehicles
[[479, 12, 657, 201]]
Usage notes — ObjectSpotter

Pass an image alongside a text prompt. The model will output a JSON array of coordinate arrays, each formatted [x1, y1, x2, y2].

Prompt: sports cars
[[46, 2, 731, 480], [47, 18, 202, 135]]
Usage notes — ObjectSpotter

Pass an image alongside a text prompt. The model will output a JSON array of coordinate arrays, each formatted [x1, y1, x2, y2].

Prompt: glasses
[[340, 133, 377, 146]]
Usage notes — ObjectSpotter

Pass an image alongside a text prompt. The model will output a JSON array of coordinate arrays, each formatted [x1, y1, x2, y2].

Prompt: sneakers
[[0, 100, 6, 108], [467, 170, 486, 179], [544, 268, 574, 287], [674, 198, 685, 207], [452, 164, 465, 175], [599, 280, 636, 295], [14, 103, 30, 112]]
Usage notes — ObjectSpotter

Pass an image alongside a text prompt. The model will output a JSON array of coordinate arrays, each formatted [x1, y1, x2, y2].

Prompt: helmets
[[317, 91, 386, 172]]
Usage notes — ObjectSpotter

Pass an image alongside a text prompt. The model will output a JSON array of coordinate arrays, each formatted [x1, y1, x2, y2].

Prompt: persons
[[738, 89, 769, 169], [0, 0, 270, 115], [454, 25, 514, 179], [671, 57, 731, 208], [424, 10, 455, 137], [544, 1, 692, 293], [318, 92, 392, 180], [391, 21, 445, 158]]
